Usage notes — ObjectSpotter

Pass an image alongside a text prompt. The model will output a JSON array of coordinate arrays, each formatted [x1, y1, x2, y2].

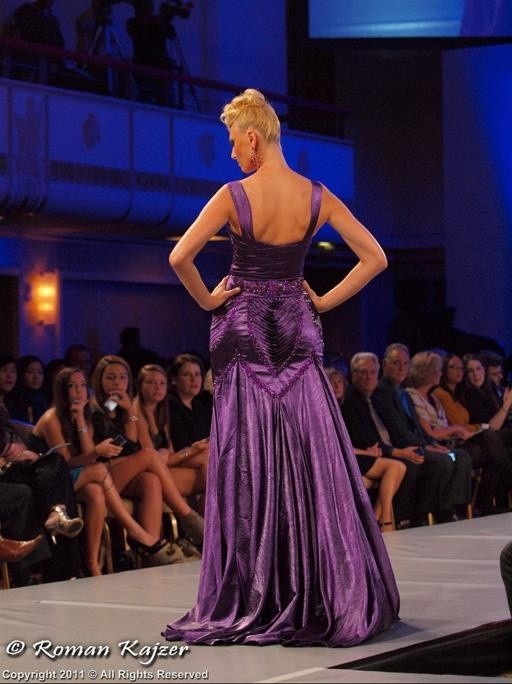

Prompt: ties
[[366, 397, 393, 447], [398, 391, 422, 433]]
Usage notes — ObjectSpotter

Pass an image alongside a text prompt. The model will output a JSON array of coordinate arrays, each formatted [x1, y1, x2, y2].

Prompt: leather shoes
[[0, 535, 45, 562]]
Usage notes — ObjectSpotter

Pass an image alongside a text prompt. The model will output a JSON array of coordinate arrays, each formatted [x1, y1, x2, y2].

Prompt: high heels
[[126, 535, 168, 554], [178, 509, 204, 546], [44, 504, 83, 544]]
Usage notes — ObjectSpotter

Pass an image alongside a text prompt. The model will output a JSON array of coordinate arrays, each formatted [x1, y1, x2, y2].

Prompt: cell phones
[[104, 395, 120, 411], [415, 447, 424, 456], [110, 434, 126, 447]]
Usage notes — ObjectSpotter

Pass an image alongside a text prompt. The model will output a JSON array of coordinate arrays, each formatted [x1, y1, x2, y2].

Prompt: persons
[[12, 0, 176, 105], [325, 343, 512, 532], [160, 89, 400, 648], [0, 326, 213, 588]]
